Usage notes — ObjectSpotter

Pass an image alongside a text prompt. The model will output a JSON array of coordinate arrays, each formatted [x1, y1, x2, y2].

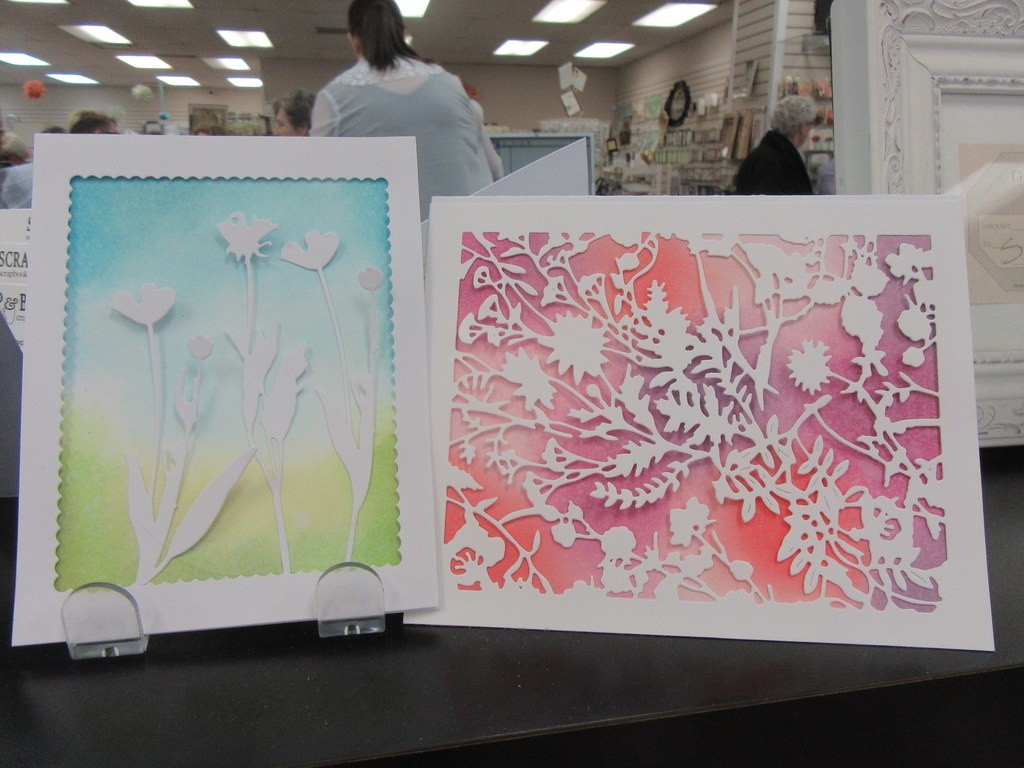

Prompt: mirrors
[[665, 80, 692, 126]]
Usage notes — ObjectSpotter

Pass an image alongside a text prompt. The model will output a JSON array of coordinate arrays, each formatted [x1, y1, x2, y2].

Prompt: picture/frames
[[829, 0, 1024, 448], [719, 109, 752, 160]]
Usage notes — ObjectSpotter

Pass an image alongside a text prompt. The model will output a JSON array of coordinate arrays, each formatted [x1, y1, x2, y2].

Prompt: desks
[[1, 445, 1024, 768]]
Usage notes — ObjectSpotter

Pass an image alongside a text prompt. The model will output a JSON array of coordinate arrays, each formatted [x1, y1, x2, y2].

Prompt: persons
[[817, 157, 836, 196], [734, 95, 817, 195], [306, 0, 504, 223], [0, 88, 317, 170]]
